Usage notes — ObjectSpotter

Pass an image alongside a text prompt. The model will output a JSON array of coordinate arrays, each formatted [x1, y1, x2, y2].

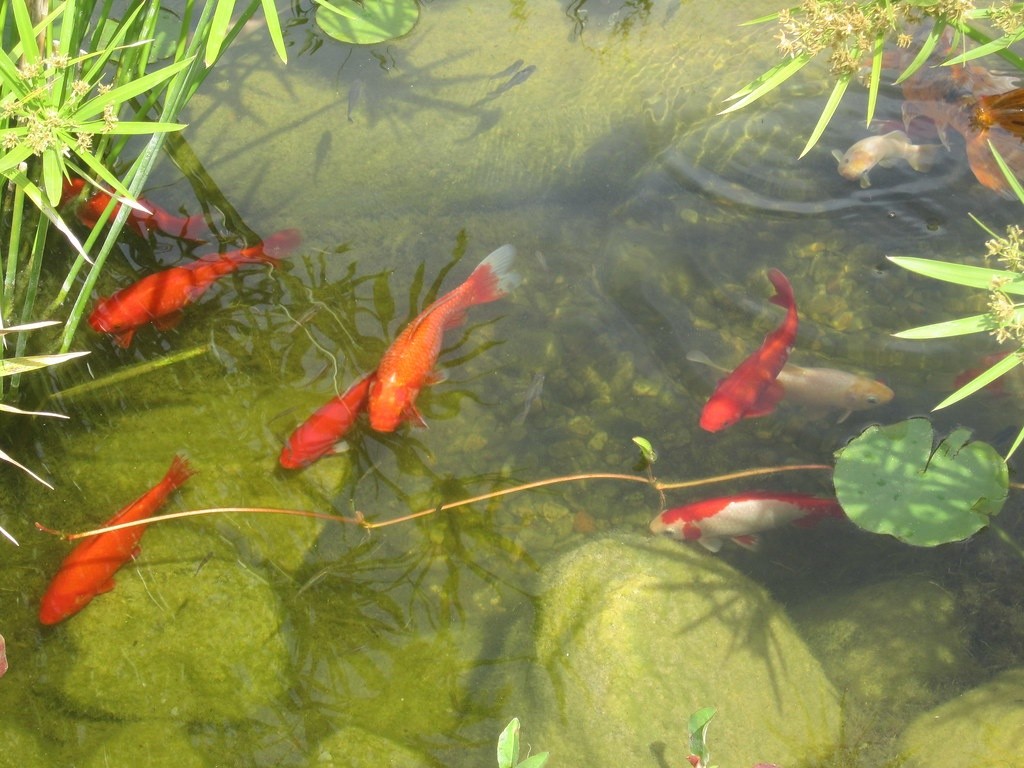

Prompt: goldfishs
[[40, 454, 195, 624], [830, 131, 942, 189], [682, 270, 894, 433], [650, 489, 842, 554], [90, 229, 303, 348], [64, 179, 208, 243], [280, 243, 520, 470], [948, 351, 1023, 403]]
